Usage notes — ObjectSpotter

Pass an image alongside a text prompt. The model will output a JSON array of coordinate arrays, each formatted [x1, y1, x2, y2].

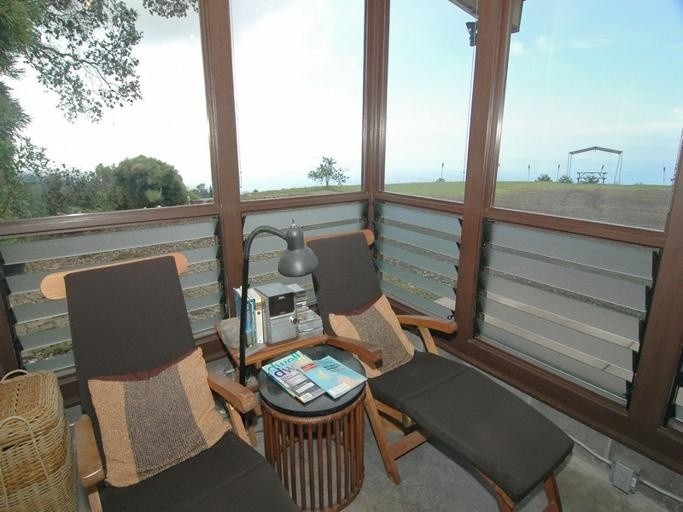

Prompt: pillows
[[85, 347, 231, 487], [328, 293, 414, 381]]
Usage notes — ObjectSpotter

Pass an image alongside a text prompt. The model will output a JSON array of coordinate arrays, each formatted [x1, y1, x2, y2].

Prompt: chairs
[[304, 228, 576, 512], [38, 252, 307, 512]]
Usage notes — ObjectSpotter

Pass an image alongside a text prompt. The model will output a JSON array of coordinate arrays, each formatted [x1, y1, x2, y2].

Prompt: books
[[297, 355, 368, 399], [233, 284, 322, 349], [261, 350, 314, 399], [268, 354, 326, 406]]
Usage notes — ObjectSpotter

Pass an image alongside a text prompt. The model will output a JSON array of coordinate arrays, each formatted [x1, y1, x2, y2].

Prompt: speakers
[[254, 282, 298, 344]]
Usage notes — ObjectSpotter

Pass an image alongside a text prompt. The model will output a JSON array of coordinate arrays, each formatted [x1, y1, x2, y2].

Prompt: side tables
[[257, 343, 368, 511], [213, 318, 328, 447]]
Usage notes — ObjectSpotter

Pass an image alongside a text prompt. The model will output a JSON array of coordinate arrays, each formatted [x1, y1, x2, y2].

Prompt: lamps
[[240, 224, 318, 429]]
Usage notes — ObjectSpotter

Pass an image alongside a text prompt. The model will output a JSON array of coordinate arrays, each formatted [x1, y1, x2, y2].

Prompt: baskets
[[1, 370, 77, 512]]
